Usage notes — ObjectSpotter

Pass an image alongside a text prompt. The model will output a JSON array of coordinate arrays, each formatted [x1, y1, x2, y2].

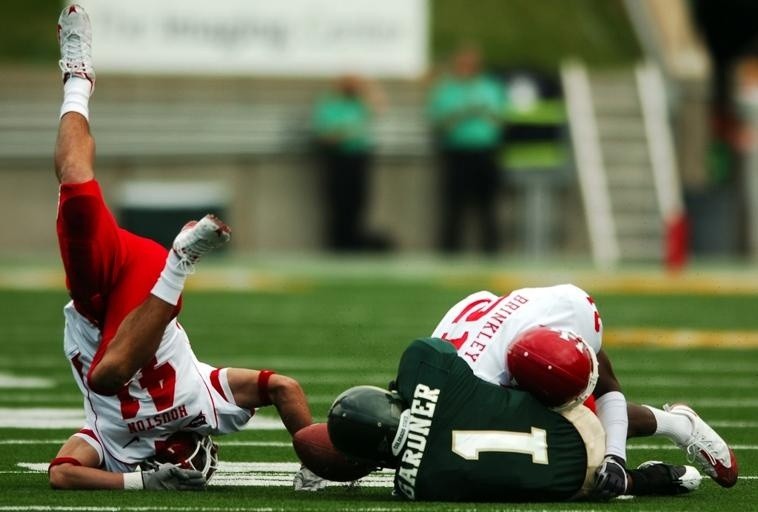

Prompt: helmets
[[143, 430, 219, 487], [507, 328, 599, 414], [327, 385, 411, 470]]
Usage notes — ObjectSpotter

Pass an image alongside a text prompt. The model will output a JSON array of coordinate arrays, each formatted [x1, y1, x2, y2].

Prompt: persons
[[423, 43, 512, 267], [327, 338, 702, 502], [427, 285, 739, 499], [49, 3, 325, 491], [309, 72, 394, 255]]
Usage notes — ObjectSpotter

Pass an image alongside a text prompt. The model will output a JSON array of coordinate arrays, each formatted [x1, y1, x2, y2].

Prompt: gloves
[[293, 464, 326, 491], [123, 463, 206, 492], [592, 456, 629, 500]]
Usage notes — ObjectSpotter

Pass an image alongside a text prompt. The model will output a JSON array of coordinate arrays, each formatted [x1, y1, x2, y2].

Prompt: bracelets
[[122, 470, 144, 492]]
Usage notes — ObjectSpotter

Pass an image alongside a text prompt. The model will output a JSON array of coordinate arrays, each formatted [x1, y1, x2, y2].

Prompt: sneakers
[[173, 214, 232, 265], [662, 402, 738, 487], [58, 4, 95, 96], [638, 461, 702, 493]]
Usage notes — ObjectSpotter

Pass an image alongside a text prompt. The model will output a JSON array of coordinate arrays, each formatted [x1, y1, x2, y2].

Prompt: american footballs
[[294, 423, 374, 481]]
